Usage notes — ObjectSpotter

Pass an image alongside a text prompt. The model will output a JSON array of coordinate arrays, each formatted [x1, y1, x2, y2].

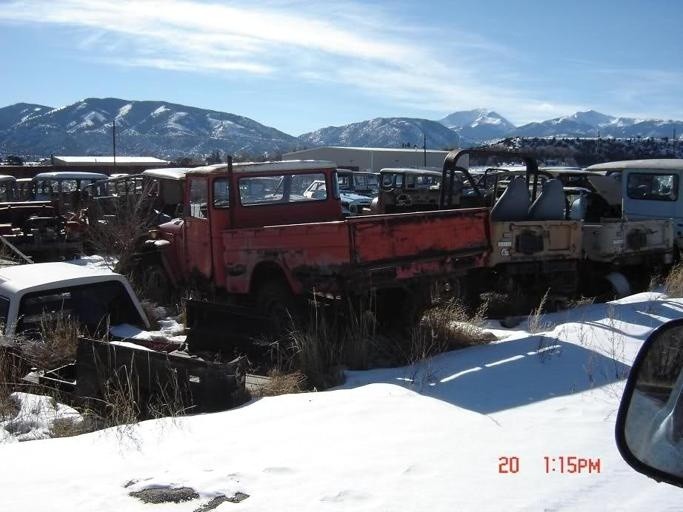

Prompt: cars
[[637, 370, 682, 479], [0, 145, 683, 319]]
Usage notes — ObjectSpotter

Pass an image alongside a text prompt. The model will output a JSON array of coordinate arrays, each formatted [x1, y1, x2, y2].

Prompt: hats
[[637, 370, 682, 479]]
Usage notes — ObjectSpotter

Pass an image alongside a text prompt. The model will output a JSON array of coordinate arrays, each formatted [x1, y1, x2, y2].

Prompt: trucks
[[2, 260, 250, 421], [114, 157, 495, 363]]
